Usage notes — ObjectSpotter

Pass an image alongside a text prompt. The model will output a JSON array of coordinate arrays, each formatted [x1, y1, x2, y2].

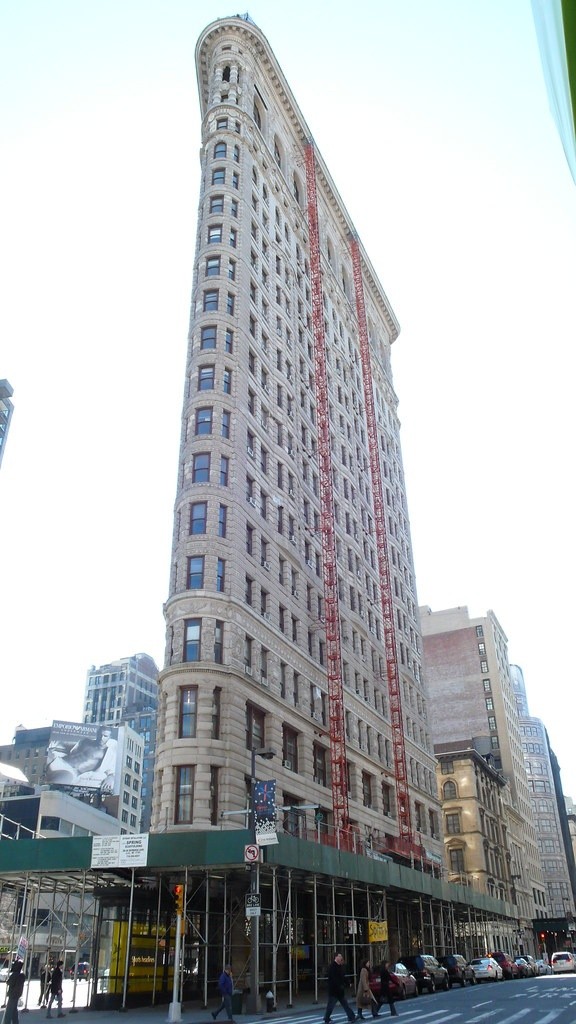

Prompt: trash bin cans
[[231, 992, 243, 1016]]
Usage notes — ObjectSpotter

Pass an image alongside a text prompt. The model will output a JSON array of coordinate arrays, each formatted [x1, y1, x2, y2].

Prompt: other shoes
[[374, 1014, 381, 1018], [357, 1015, 365, 1019], [350, 1016, 359, 1023], [212, 1012, 216, 1021], [325, 1019, 333, 1024], [391, 1013, 399, 1017], [58, 1013, 66, 1018], [47, 1015, 53, 1018]]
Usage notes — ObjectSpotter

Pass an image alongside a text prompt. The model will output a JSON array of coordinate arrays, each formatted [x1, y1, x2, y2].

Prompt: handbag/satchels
[[360, 991, 371, 1005]]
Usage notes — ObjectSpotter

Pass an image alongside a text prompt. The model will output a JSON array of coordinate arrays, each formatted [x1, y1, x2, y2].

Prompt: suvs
[[434, 954, 477, 987], [483, 950, 521, 980], [396, 954, 451, 994], [69, 961, 101, 981]]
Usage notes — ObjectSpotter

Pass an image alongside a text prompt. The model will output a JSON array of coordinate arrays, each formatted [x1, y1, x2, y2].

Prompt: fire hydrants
[[265, 990, 274, 1013]]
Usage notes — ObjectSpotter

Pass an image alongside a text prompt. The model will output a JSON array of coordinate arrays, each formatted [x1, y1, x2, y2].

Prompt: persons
[[372, 960, 400, 1018], [355, 958, 378, 1019], [37, 965, 55, 1006], [46, 960, 66, 1018], [4, 960, 26, 1024], [322, 953, 361, 1024], [211, 964, 233, 1020], [45, 725, 117, 790]]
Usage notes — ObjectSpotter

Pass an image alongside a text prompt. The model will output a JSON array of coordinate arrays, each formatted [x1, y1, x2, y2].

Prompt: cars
[[470, 957, 504, 984], [0, 967, 24, 982], [368, 962, 419, 1003], [514, 954, 552, 978]]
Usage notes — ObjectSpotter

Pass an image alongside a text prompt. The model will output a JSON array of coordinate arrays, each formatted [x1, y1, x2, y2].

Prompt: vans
[[550, 951, 576, 974]]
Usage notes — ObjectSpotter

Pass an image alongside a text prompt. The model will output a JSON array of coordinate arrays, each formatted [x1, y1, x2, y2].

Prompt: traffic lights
[[173, 884, 185, 915], [540, 933, 546, 942], [180, 918, 189, 935], [537, 944, 543, 951]]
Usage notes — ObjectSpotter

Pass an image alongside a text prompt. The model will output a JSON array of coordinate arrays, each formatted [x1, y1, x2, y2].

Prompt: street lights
[[248, 747, 277, 1015]]
